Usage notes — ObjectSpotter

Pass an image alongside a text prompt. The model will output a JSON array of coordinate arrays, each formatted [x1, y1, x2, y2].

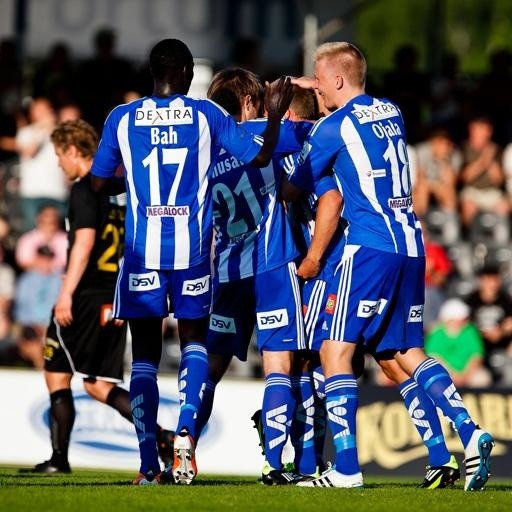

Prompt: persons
[[188, 66, 314, 485], [425, 296, 491, 389], [1, 212, 17, 340], [0, 315, 44, 369], [19, 118, 174, 477], [89, 38, 296, 487], [16, 100, 73, 235], [16, 204, 69, 343], [262, 81, 460, 489], [502, 143, 512, 195], [415, 133, 463, 219], [458, 119, 508, 227], [299, 41, 495, 491], [459, 263, 512, 388], [58, 102, 80, 123]]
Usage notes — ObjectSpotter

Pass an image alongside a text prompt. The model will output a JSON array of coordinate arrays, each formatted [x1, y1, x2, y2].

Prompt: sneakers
[[417, 455, 460, 491], [261, 469, 316, 488], [157, 428, 175, 468], [171, 431, 197, 485], [131, 474, 169, 489], [296, 463, 363, 490], [18, 459, 71, 475], [462, 423, 494, 492]]
